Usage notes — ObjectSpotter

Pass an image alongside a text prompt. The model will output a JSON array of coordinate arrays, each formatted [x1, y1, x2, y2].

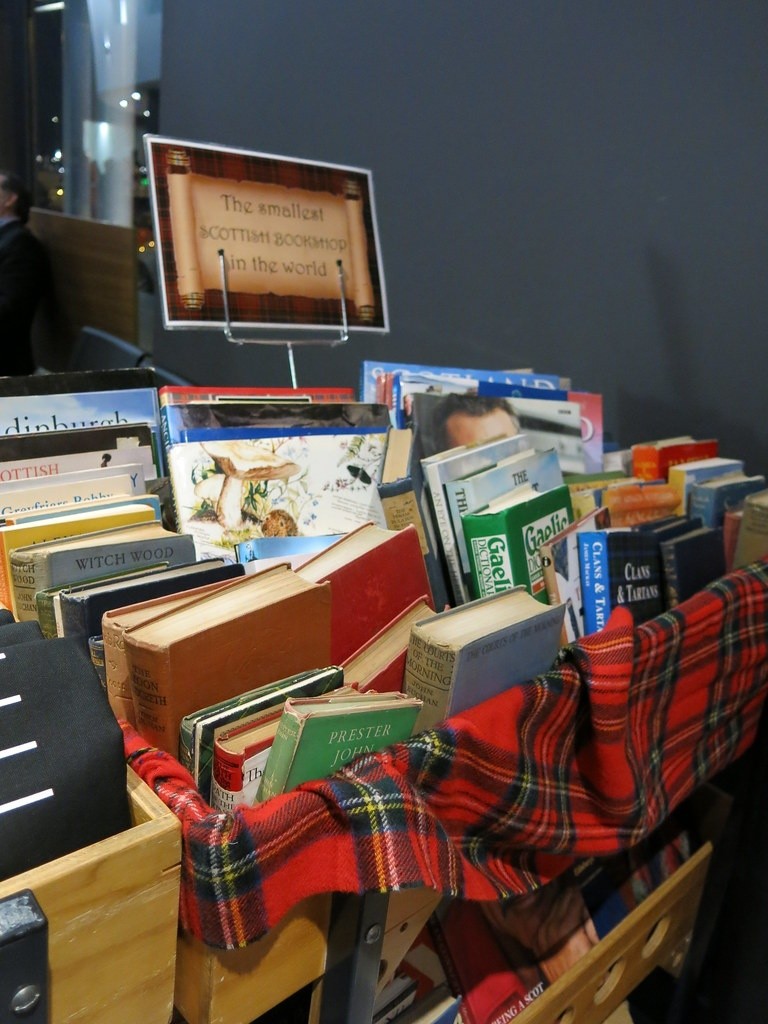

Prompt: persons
[[427, 388, 536, 455], [1, 160, 59, 383]]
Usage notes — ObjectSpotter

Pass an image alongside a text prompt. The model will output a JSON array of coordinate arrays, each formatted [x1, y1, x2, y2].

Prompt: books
[[2, 356, 768, 815]]
